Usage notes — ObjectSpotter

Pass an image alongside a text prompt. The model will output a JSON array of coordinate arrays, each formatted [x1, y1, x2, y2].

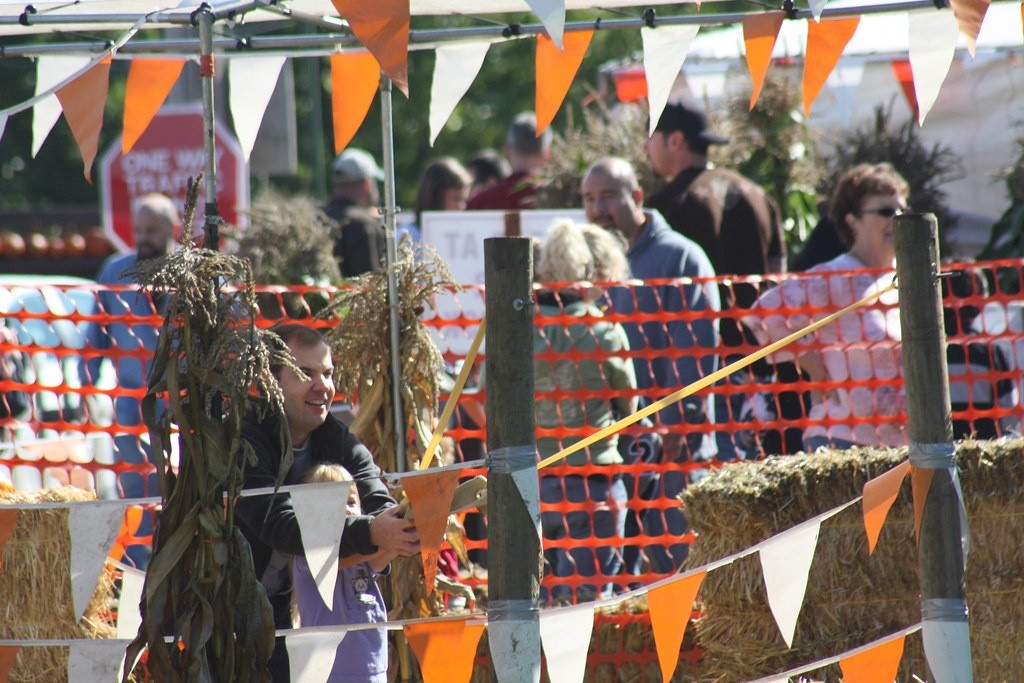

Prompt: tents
[[601, 0, 1024, 257]]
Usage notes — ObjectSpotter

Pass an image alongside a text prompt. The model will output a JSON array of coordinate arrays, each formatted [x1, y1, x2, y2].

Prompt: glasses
[[852, 206, 911, 217]]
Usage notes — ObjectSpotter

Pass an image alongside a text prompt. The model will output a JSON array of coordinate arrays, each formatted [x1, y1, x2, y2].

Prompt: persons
[[464, 147, 511, 193], [292, 460, 391, 683], [639, 93, 790, 460], [222, 322, 425, 683], [578, 155, 725, 576], [939, 254, 1023, 440], [397, 157, 475, 275], [76, 188, 252, 574], [530, 218, 643, 603], [319, 146, 388, 278], [464, 107, 558, 209], [742, 159, 921, 452]]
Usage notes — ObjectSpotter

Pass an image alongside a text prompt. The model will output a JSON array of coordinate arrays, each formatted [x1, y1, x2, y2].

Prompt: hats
[[333, 146, 386, 181], [645, 94, 730, 142]]
